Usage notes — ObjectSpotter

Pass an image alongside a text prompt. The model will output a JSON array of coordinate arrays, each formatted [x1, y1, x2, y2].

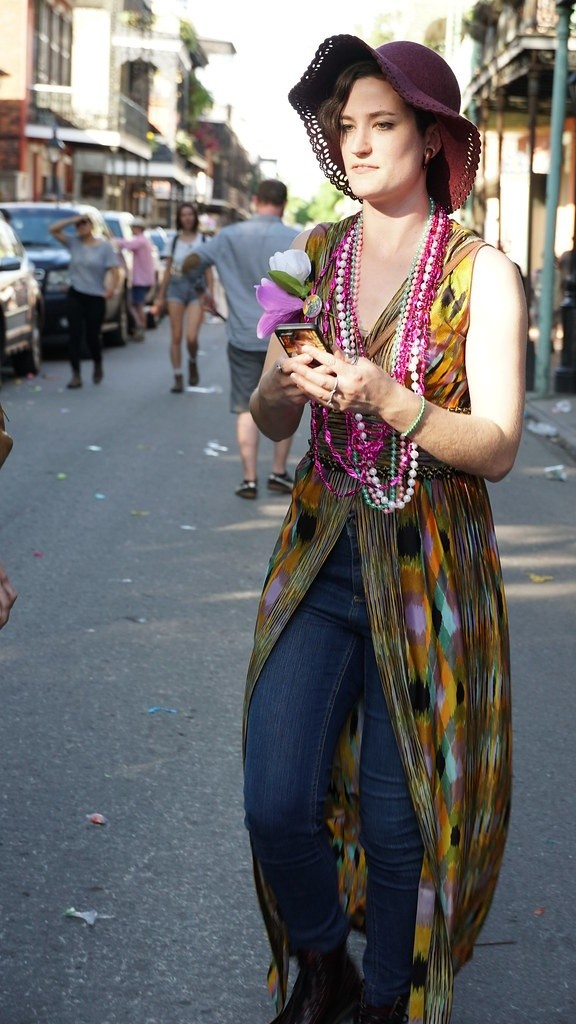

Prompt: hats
[[129, 218, 146, 229], [288, 33, 481, 214]]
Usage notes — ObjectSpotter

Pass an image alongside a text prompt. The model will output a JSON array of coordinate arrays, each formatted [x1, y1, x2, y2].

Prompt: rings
[[328, 392, 337, 402], [333, 378, 339, 391], [275, 363, 282, 372], [349, 355, 360, 365]]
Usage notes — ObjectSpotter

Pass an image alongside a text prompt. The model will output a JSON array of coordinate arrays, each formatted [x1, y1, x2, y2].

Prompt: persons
[[245, 32, 527, 1024], [51, 214, 120, 388], [180, 179, 304, 498], [152, 205, 215, 392], [113, 218, 154, 342]]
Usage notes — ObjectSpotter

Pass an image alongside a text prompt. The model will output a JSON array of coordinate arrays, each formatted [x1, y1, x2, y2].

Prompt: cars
[[0, 209, 45, 376], [146, 227, 170, 272], [102, 211, 153, 338]]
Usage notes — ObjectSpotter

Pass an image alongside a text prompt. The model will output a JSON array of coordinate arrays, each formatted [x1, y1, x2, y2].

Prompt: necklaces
[[304, 194, 451, 513]]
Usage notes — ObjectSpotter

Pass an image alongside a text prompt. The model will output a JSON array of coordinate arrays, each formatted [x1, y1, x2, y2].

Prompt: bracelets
[[400, 394, 427, 438]]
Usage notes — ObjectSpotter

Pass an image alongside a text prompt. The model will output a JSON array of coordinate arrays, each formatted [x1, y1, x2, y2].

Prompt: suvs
[[0, 202, 131, 349]]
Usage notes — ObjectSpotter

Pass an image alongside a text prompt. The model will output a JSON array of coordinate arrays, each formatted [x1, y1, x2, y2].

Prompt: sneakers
[[236, 480, 257, 498], [267, 472, 294, 493]]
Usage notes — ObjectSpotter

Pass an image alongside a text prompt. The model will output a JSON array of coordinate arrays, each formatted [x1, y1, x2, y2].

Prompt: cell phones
[[275, 323, 337, 377]]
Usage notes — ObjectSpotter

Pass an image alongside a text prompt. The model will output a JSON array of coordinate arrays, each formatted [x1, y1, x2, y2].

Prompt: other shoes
[[93, 363, 103, 382], [67, 378, 82, 388]]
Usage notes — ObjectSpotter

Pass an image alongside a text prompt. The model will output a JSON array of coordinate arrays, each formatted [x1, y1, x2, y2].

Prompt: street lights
[[254, 157, 277, 190]]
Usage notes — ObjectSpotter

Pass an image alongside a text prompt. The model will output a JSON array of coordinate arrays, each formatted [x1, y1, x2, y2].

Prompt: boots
[[354, 981, 410, 1023], [268, 943, 359, 1024], [171, 375, 182, 391], [189, 360, 198, 385]]
